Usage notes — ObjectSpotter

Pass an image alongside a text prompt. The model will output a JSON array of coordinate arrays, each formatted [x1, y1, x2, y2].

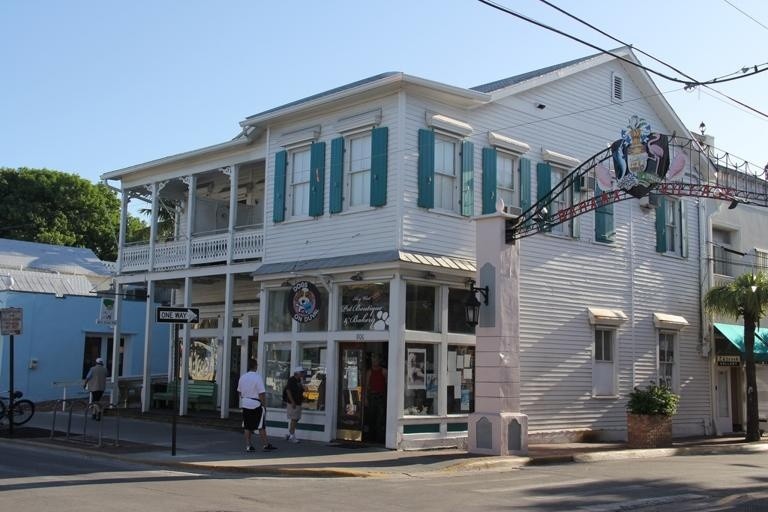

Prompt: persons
[[283, 366, 308, 443], [237, 359, 280, 452], [83, 358, 108, 420], [364, 352, 387, 442]]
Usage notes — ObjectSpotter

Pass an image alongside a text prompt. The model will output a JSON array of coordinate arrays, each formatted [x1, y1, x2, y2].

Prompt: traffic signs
[[156, 306, 200, 324]]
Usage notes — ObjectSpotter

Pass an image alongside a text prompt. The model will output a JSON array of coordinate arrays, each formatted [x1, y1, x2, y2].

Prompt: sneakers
[[246, 445, 256, 451], [289, 435, 300, 442], [263, 444, 281, 452], [91, 411, 101, 421]]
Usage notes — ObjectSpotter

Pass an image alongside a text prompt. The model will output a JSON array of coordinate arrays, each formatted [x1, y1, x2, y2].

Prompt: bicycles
[[1, 391, 34, 426]]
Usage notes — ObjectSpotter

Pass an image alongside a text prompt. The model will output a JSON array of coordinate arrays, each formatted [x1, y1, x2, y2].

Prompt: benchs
[[152, 380, 220, 414]]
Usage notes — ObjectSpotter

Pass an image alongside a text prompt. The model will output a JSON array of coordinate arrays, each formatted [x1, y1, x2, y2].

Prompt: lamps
[[460, 277, 490, 327]]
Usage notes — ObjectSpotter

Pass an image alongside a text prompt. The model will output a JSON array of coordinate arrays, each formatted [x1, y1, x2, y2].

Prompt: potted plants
[[625, 382, 682, 447]]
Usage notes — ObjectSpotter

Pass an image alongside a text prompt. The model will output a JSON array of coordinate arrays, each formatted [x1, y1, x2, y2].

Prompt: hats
[[295, 367, 304, 371], [96, 358, 104, 365]]
